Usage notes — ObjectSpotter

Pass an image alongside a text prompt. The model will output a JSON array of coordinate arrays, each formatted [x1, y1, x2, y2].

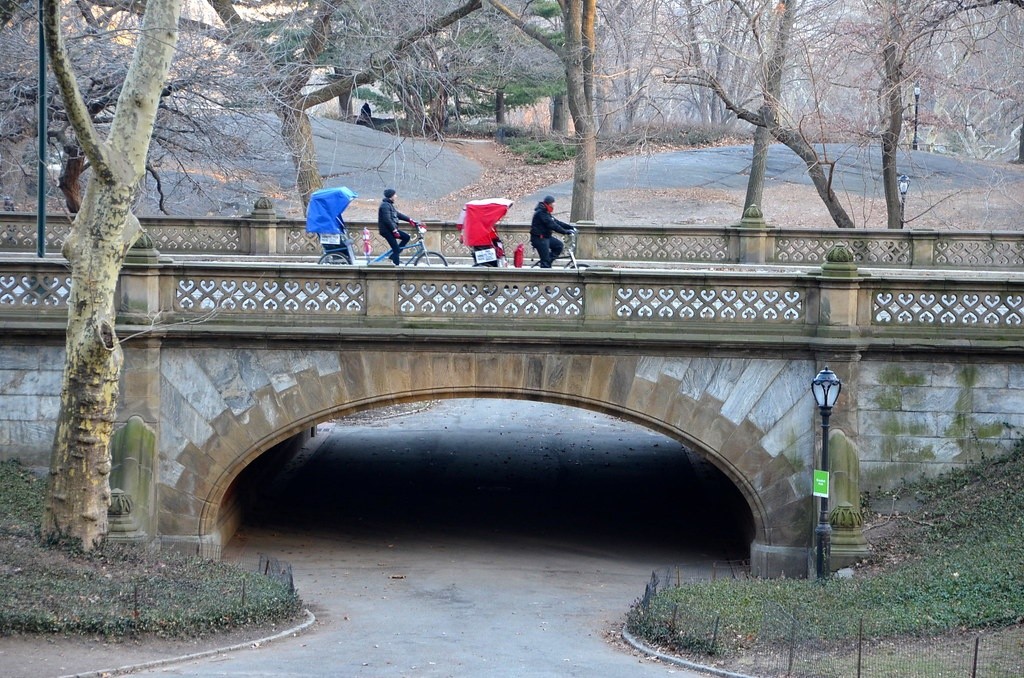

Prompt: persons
[[378, 189, 415, 265], [530, 196, 577, 268]]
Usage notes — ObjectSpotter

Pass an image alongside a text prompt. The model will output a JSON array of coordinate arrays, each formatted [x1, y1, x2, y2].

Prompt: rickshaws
[[456, 198, 591, 269], [306, 186, 449, 268]]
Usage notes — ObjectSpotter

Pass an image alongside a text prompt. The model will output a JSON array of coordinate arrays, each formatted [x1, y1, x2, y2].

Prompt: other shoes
[[396, 261, 406, 267]]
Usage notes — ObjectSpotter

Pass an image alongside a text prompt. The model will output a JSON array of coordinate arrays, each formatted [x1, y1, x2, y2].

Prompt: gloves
[[567, 229, 575, 235], [393, 230, 400, 238], [407, 217, 416, 227]]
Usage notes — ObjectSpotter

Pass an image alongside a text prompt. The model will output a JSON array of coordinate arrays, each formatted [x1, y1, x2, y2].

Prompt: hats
[[384, 189, 396, 197], [545, 196, 554, 204]]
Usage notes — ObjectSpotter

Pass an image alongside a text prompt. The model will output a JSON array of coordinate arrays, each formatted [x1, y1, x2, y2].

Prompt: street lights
[[898, 174, 911, 229], [912, 82, 921, 150], [811, 366, 842, 579]]
